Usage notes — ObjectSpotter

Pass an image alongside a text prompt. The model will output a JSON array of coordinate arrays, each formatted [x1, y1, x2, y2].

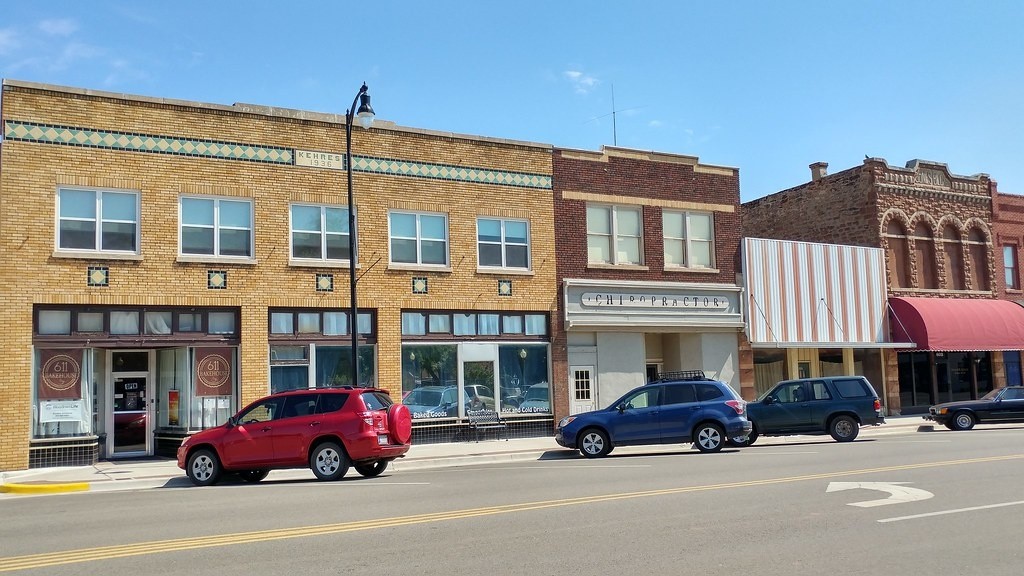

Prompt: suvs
[[177, 385, 412, 486], [556, 369, 752, 458], [728, 375, 885, 443], [402, 386, 471, 419]]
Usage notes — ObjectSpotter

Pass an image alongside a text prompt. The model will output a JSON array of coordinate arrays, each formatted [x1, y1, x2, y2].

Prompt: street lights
[[345, 80, 375, 388]]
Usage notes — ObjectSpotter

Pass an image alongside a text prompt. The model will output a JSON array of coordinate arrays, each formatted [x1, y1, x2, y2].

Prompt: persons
[[793, 387, 804, 401]]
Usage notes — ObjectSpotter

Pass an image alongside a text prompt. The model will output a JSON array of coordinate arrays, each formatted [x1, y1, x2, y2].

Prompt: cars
[[115, 413, 145, 443], [519, 383, 549, 411], [923, 386, 1024, 430], [465, 384, 503, 412]]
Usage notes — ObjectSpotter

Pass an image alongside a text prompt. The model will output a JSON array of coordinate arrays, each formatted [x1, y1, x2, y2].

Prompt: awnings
[[888, 296, 1024, 352], [751, 293, 917, 349]]
